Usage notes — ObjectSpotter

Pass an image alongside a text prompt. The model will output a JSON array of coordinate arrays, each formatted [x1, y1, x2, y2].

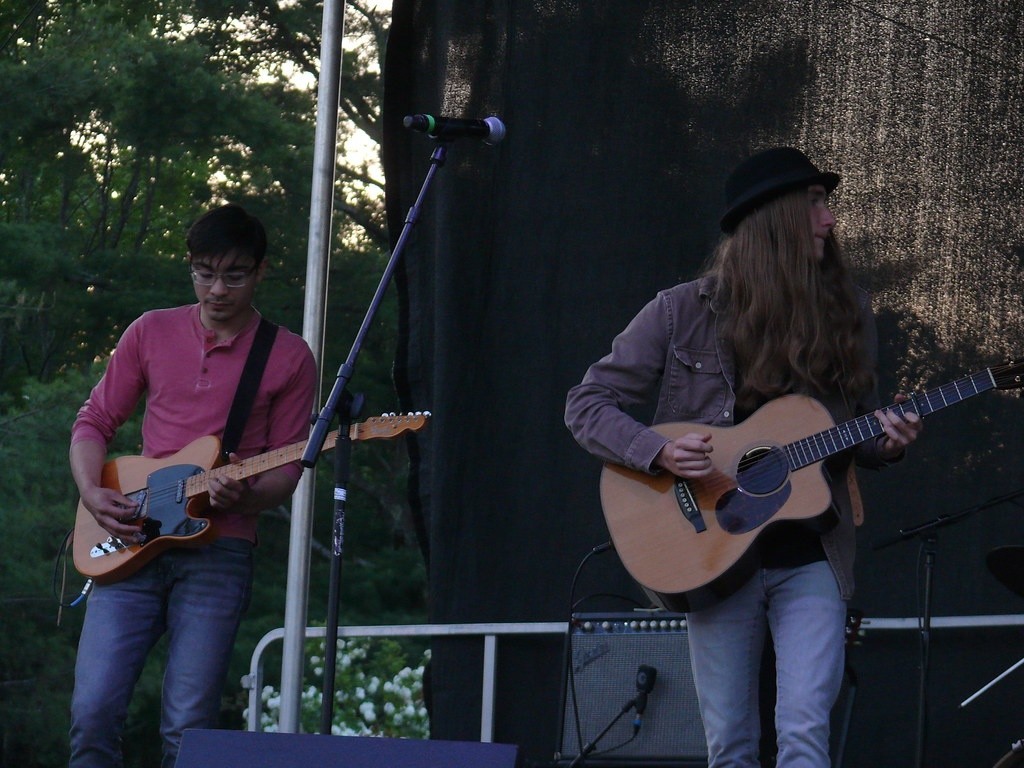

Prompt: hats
[[719, 146, 841, 234]]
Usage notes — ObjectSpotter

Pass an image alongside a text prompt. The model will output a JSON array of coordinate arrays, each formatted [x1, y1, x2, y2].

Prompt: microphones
[[403, 114, 507, 146], [632, 664, 658, 734]]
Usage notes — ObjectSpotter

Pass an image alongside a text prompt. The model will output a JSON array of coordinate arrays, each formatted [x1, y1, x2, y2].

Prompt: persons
[[69, 204, 317, 768], [565, 147, 922, 768]]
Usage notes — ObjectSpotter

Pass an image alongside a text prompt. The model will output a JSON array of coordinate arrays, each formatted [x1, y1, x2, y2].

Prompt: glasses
[[189, 259, 256, 288]]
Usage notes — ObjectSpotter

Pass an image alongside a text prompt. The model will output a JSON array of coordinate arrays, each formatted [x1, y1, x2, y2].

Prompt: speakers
[[172, 729, 522, 768], [552, 611, 711, 762]]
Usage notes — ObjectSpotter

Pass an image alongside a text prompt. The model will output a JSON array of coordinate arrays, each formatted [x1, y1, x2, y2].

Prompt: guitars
[[596, 358, 1024, 613], [70, 404, 437, 586]]
